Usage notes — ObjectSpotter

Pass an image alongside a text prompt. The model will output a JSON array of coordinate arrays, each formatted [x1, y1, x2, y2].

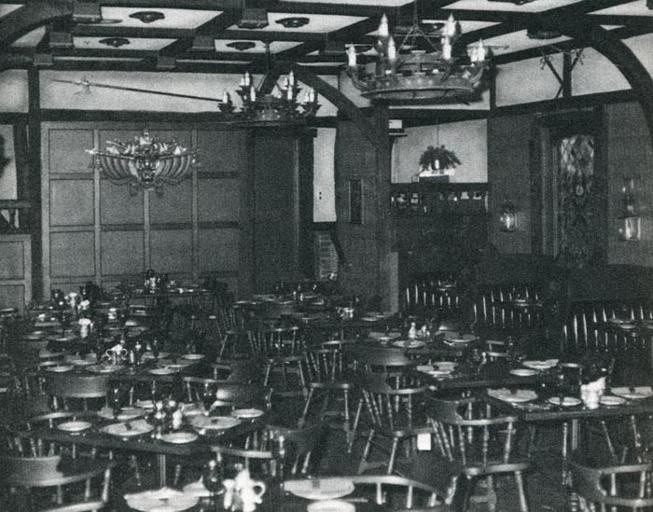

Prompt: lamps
[[342, 2, 500, 107], [216, 40, 319, 130], [418, 122, 462, 178]]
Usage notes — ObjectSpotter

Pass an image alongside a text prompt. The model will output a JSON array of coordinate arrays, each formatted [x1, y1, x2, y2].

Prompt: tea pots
[[223, 470, 266, 507]]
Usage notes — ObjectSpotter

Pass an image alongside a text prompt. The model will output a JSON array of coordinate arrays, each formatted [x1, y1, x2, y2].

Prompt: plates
[[285, 477, 355, 512], [123, 479, 220, 511], [366, 326, 479, 348], [48, 346, 205, 377], [487, 376, 651, 407], [407, 347, 556, 379], [61, 398, 262, 446]]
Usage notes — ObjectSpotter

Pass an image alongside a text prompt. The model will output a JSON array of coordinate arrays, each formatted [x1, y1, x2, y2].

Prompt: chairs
[[0, 270, 653, 512]]
